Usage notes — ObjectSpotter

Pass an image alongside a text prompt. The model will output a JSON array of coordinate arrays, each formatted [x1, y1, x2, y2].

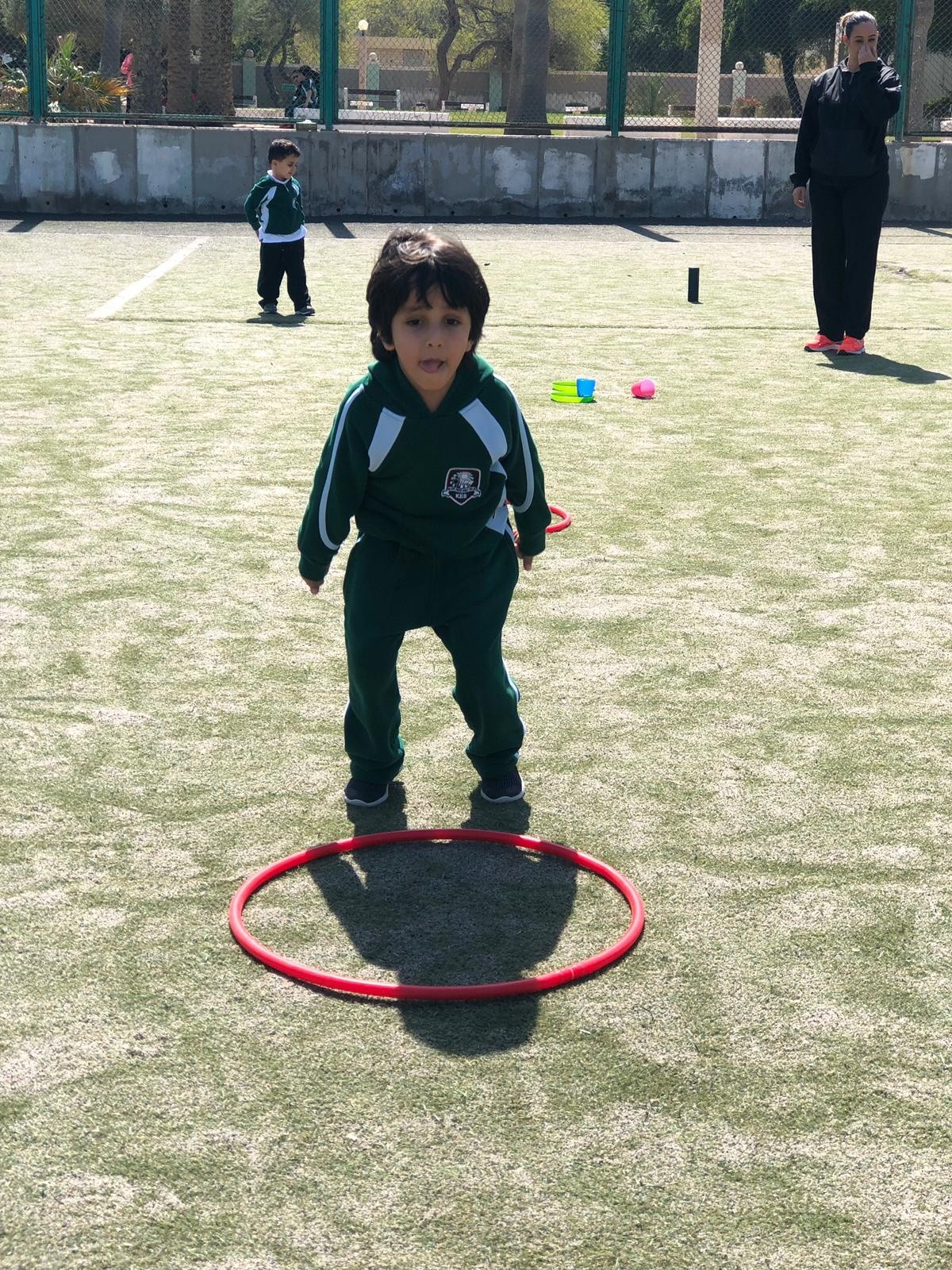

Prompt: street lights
[[358, 21, 368, 110]]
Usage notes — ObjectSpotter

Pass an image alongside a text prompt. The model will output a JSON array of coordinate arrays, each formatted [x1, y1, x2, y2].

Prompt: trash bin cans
[[923, 104, 941, 132]]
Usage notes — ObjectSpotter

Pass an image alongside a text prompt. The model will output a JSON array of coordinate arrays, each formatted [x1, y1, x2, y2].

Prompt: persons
[[789, 11, 902, 355], [286, 65, 319, 129], [298, 227, 548, 807], [244, 140, 315, 315], [121, 40, 133, 126]]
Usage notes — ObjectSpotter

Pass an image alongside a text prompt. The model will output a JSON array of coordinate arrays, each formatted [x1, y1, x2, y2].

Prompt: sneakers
[[803, 333, 843, 352], [837, 335, 865, 355]]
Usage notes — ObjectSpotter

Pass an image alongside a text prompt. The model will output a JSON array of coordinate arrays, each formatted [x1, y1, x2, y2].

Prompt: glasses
[[292, 74, 302, 79]]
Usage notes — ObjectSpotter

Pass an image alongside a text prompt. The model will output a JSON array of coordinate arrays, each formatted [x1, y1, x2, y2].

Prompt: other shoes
[[279, 124, 292, 128], [263, 303, 277, 313], [481, 765, 524, 803], [343, 762, 403, 806], [295, 305, 316, 315]]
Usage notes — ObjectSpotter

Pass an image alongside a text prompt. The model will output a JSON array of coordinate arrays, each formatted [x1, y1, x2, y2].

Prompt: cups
[[631, 380, 655, 398], [576, 378, 595, 397]]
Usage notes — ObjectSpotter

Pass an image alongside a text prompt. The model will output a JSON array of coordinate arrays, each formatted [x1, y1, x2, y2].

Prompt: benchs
[[668, 105, 695, 117], [441, 100, 490, 113], [344, 87, 401, 111]]
